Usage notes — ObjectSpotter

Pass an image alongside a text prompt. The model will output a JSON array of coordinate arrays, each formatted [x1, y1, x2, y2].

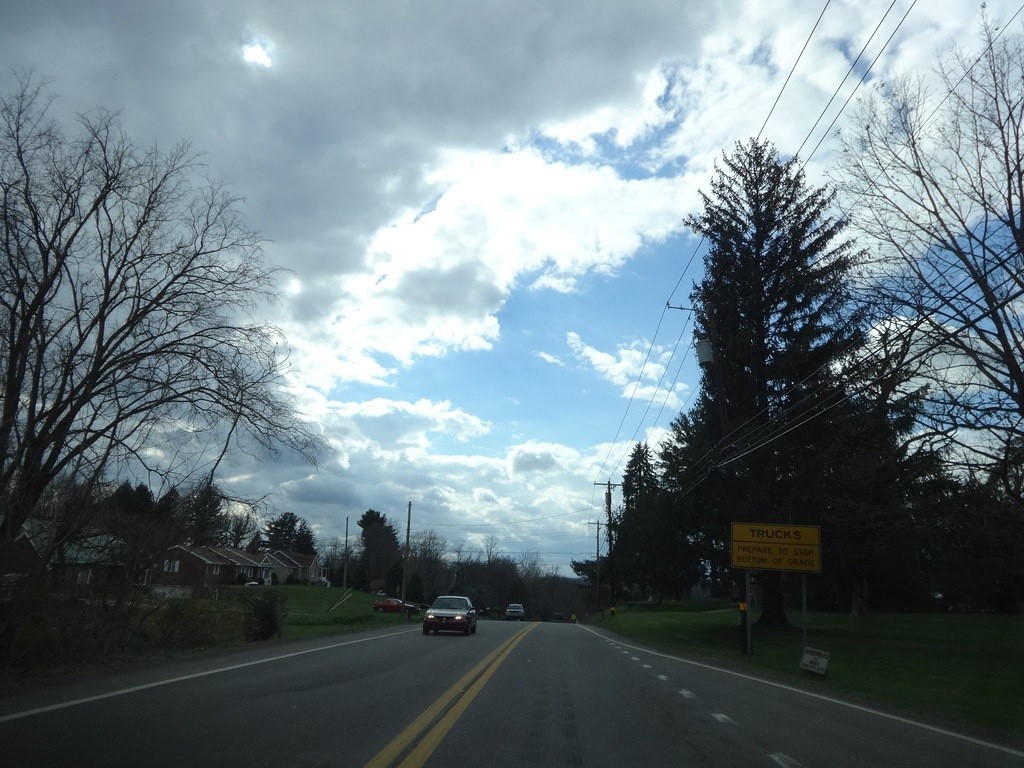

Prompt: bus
[[551, 612, 563, 622]]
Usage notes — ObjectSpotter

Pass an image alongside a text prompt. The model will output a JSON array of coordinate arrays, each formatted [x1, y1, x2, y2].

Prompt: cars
[[423, 596, 477, 636], [505, 603, 525, 620], [373, 599, 414, 612]]
